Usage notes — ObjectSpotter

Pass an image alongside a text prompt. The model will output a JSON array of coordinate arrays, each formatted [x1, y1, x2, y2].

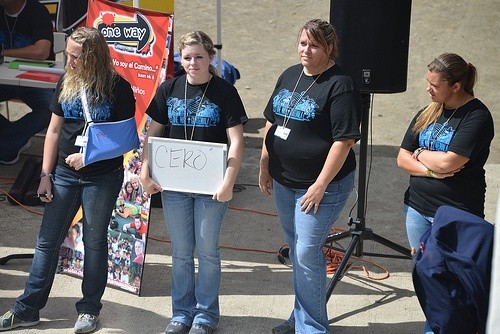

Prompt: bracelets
[[429, 170, 433, 177]]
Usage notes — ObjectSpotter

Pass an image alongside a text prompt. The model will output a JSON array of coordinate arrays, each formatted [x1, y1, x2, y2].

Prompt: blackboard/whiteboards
[[147, 136, 227, 196]]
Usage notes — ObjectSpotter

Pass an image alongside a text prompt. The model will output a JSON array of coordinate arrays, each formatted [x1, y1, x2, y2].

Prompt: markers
[[32, 194, 54, 198]]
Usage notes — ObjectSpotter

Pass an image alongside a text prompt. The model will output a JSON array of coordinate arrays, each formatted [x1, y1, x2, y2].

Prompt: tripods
[[282, 99, 411, 303]]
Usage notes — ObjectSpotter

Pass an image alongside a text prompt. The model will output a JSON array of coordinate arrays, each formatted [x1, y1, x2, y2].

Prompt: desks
[[0, 54, 65, 88]]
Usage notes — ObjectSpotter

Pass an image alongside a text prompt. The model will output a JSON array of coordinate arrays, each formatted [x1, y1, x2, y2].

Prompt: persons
[[396, 52, 494, 334], [57, 119, 151, 288], [138, 31, 249, 334], [259, 19, 360, 334], [0, 0, 56, 165], [0, 26, 136, 333]]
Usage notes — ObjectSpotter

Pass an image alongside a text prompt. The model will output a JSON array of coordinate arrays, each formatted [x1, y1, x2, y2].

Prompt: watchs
[[413, 147, 425, 159]]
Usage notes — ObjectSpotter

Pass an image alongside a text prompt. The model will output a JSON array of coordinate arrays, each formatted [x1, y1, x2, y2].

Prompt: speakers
[[330, 0, 411, 95]]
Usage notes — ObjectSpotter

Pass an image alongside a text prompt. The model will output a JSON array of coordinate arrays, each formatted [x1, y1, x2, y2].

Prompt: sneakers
[[164, 320, 189, 334], [189, 323, 213, 334], [0, 311, 39, 331], [73, 312, 98, 334], [272, 322, 295, 334], [0, 137, 30, 165]]
